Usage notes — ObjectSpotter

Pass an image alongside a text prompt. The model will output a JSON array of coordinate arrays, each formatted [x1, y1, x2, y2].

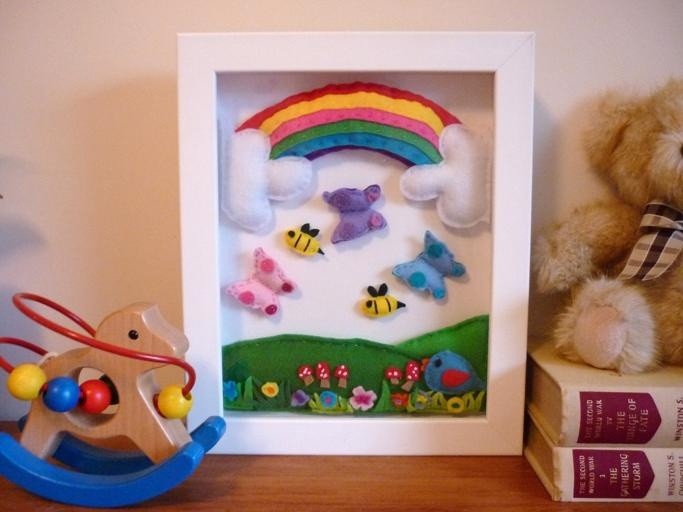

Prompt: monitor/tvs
[[521, 334, 683, 503]]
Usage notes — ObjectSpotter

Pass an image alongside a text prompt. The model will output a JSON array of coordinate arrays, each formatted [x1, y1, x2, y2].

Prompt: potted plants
[[173, 27, 539, 458]]
